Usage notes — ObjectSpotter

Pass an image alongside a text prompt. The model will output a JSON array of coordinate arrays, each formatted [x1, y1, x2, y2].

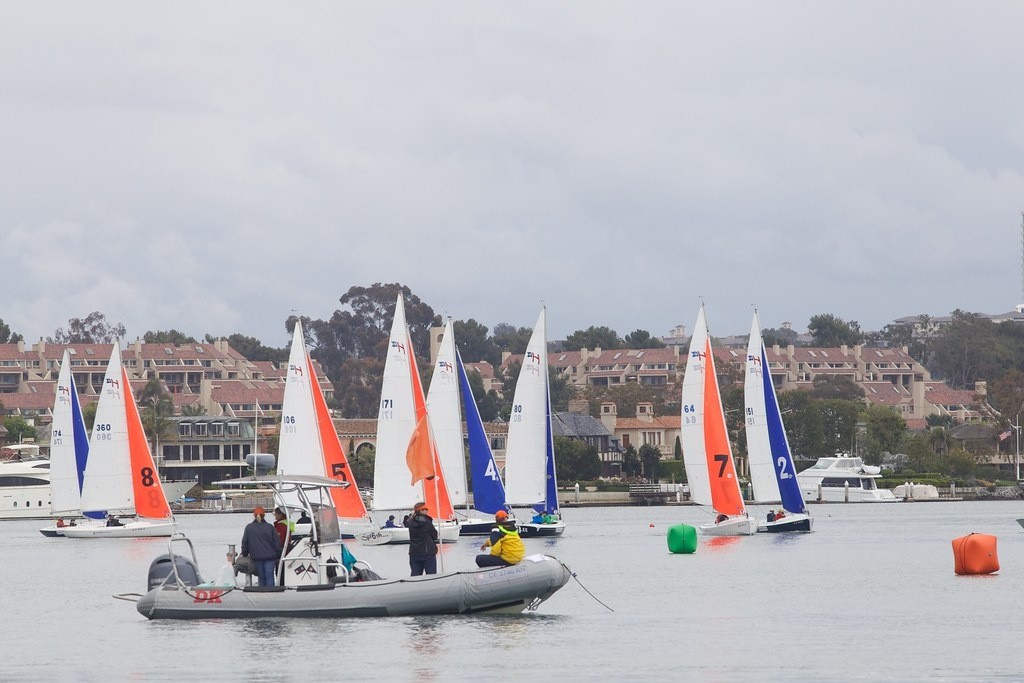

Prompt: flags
[[342, 544, 356, 575], [406, 410, 435, 486], [999, 427, 1011, 441]]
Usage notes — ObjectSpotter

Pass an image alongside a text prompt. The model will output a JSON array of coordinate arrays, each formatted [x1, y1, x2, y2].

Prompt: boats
[[797, 453, 902, 503], [110, 474, 571, 620]]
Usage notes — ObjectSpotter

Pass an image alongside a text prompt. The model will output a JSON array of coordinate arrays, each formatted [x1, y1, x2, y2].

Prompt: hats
[[495, 510, 510, 522], [415, 503, 431, 510], [254, 507, 265, 517], [275, 506, 286, 517]]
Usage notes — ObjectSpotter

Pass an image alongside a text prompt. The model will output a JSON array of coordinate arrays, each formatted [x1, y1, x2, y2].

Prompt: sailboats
[[426, 316, 517, 536], [681, 295, 757, 536], [744, 307, 815, 532], [38, 349, 120, 537], [353, 289, 462, 544], [56, 339, 180, 538], [272, 318, 369, 541], [500, 304, 567, 537]]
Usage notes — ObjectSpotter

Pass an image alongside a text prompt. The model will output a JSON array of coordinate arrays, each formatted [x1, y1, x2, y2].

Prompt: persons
[[273, 507, 295, 586], [385, 514, 398, 528], [767, 509, 785, 522], [297, 512, 310, 523], [715, 514, 729, 524], [403, 513, 411, 528], [475, 510, 524, 568], [530, 511, 543, 524], [241, 507, 282, 587], [215, 551, 246, 587], [408, 502, 438, 576], [57, 517, 66, 528], [542, 511, 552, 524], [106, 515, 125, 527]]
